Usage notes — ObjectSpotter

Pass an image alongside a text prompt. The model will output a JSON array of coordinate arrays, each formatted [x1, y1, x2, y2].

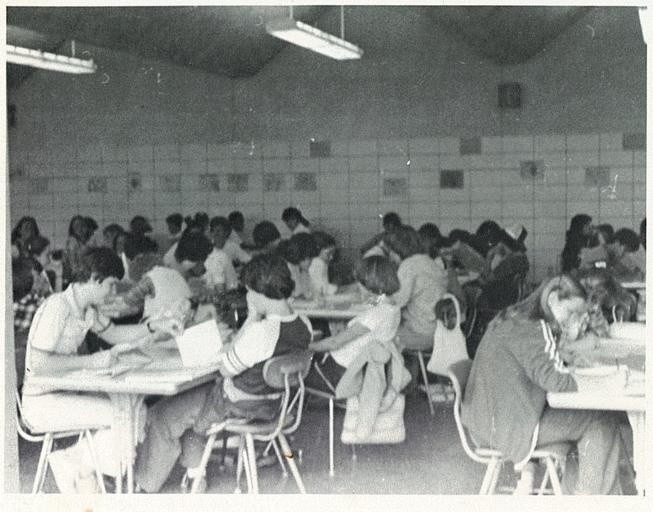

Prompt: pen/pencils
[[615, 359, 619, 370]]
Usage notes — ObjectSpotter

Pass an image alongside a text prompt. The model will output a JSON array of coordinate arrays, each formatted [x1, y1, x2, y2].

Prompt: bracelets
[[147, 324, 156, 333]]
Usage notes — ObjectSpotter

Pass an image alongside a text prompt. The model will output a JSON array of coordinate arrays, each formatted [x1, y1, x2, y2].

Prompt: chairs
[[13, 348, 108, 493], [401, 299, 463, 417], [261, 334, 402, 475], [192, 350, 313, 494], [447, 357, 572, 495], [467, 255, 528, 340]]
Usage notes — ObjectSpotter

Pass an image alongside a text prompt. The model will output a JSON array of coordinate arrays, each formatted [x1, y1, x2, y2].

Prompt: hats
[[497, 223, 528, 252]]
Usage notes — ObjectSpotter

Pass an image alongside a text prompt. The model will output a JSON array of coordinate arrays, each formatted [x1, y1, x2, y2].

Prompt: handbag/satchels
[[338, 390, 409, 449], [425, 294, 475, 383]]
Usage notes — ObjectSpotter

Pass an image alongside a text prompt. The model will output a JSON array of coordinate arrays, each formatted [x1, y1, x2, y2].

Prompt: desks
[[101, 303, 142, 319], [547, 339, 645, 496], [587, 276, 645, 290], [455, 270, 480, 286], [292, 285, 383, 318], [28, 351, 224, 493]]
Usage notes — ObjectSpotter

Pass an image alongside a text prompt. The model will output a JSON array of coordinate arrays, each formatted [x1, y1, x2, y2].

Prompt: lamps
[[265, 7, 365, 61], [6, 39, 97, 76]]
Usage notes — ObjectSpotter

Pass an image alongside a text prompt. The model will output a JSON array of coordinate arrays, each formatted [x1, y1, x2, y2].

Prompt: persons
[[307, 257, 401, 397], [422, 212, 530, 309], [562, 214, 646, 331], [461, 274, 623, 494], [387, 225, 447, 393], [124, 256, 312, 493], [21, 247, 184, 492], [8, 206, 342, 328], [359, 211, 401, 256]]
